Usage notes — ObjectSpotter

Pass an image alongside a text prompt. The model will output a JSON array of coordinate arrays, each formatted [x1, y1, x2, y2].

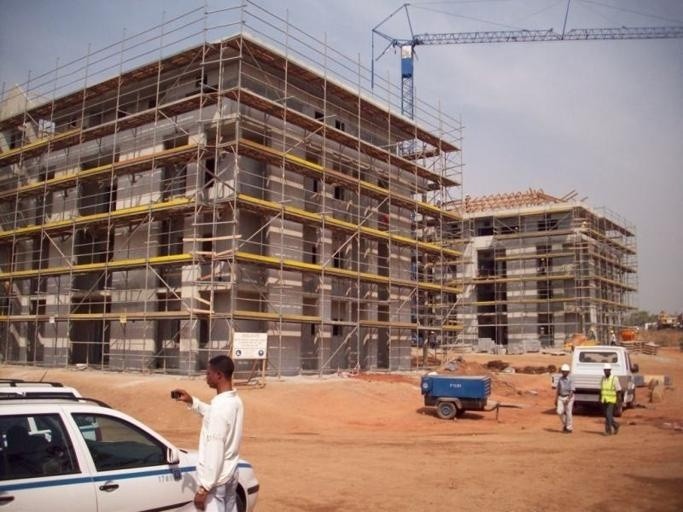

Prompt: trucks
[[551, 343, 640, 412]]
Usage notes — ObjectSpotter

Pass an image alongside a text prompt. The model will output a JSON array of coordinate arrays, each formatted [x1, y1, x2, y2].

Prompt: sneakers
[[602, 422, 619, 436], [563, 426, 572, 433]]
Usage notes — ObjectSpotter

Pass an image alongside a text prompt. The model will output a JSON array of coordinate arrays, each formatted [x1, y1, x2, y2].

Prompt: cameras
[[171, 391, 181, 398]]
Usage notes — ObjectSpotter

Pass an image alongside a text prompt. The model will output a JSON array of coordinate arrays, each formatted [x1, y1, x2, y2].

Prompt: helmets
[[603, 363, 611, 370], [610, 331, 613, 333], [560, 364, 571, 372]]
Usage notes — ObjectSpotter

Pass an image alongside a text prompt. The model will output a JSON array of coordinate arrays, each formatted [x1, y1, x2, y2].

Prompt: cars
[[0, 377, 105, 458], [408, 332, 435, 348], [0, 395, 263, 511]]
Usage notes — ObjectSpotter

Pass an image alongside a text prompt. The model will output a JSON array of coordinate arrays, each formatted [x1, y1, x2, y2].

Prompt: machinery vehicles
[[655, 309, 681, 329]]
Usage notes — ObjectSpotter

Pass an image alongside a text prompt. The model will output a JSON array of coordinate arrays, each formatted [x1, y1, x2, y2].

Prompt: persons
[[171, 355, 244, 512], [552, 364, 576, 432], [599, 364, 622, 435]]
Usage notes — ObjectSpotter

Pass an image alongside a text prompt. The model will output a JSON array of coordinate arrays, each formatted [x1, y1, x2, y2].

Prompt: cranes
[[364, 0, 683, 163]]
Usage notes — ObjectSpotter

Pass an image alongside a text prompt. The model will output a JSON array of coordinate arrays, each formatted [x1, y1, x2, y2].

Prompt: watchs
[[196, 485, 206, 496]]
